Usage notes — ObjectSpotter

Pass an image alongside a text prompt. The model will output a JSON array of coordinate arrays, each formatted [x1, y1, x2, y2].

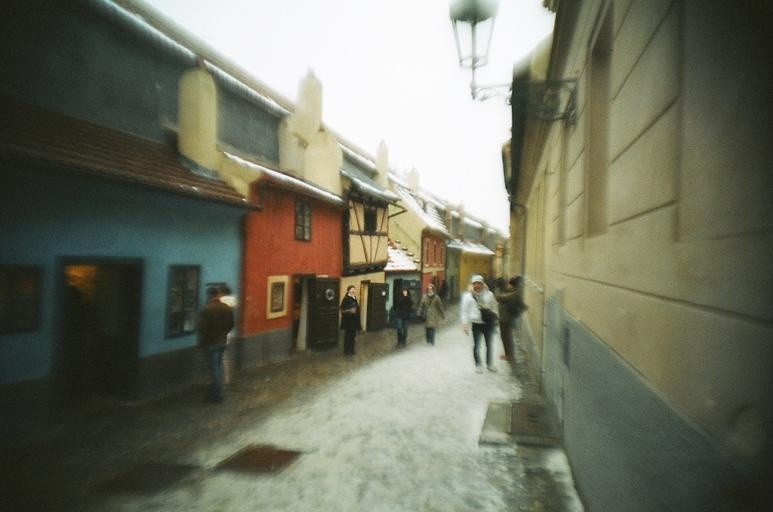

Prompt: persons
[[341, 284, 363, 358], [460, 274, 500, 372], [394, 286, 413, 347], [494, 274, 530, 361], [196, 286, 235, 403], [218, 284, 239, 396], [416, 282, 447, 346]]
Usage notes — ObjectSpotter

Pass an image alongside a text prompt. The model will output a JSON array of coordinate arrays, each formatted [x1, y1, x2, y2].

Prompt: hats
[[509, 275, 521, 288], [472, 275, 483, 285]]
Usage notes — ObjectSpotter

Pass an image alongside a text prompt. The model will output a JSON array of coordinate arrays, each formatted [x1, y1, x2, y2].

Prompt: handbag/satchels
[[389, 314, 402, 329], [421, 307, 426, 321], [507, 298, 528, 313], [481, 309, 497, 322]]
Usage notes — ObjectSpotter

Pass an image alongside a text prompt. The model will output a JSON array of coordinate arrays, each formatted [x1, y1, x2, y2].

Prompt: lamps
[[449, 0, 577, 128]]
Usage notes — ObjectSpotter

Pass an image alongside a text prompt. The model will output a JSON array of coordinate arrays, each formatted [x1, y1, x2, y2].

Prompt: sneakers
[[476, 355, 515, 373]]
